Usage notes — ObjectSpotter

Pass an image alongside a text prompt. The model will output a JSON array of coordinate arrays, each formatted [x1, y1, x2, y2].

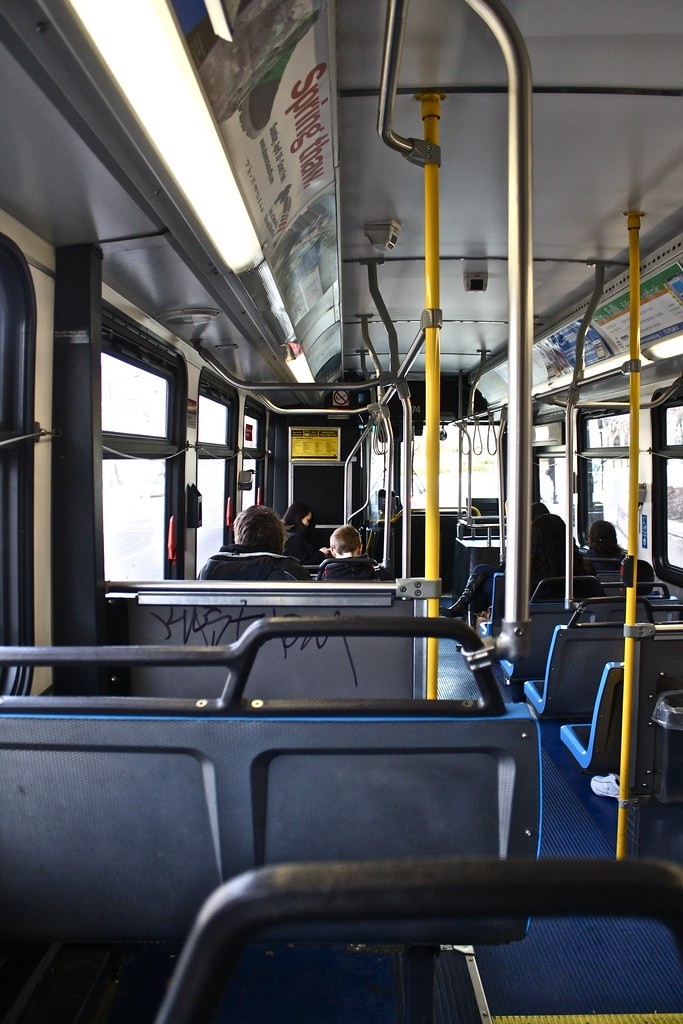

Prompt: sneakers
[[590, 774, 620, 801]]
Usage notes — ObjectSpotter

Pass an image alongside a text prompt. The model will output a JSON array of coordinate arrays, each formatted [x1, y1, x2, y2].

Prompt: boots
[[438, 572, 484, 623]]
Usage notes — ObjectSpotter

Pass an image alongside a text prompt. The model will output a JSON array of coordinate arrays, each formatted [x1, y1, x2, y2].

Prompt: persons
[[619, 560, 654, 596], [281, 501, 329, 575], [198, 505, 313, 581], [538, 343, 572, 375], [438, 500, 551, 621], [270, 211, 331, 279], [588, 521, 627, 557], [323, 525, 384, 581], [530, 515, 598, 600]]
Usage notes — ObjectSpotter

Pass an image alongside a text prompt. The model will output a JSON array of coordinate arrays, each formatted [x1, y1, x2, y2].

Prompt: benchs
[[0, 513, 683, 1024]]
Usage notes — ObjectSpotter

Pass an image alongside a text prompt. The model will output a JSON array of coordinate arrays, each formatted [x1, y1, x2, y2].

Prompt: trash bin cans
[[652, 689, 683, 804], [588, 502, 604, 538]]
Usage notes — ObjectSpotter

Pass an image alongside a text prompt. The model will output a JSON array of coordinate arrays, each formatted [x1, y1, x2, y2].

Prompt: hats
[[589, 521, 616, 543], [619, 559, 654, 595]]
